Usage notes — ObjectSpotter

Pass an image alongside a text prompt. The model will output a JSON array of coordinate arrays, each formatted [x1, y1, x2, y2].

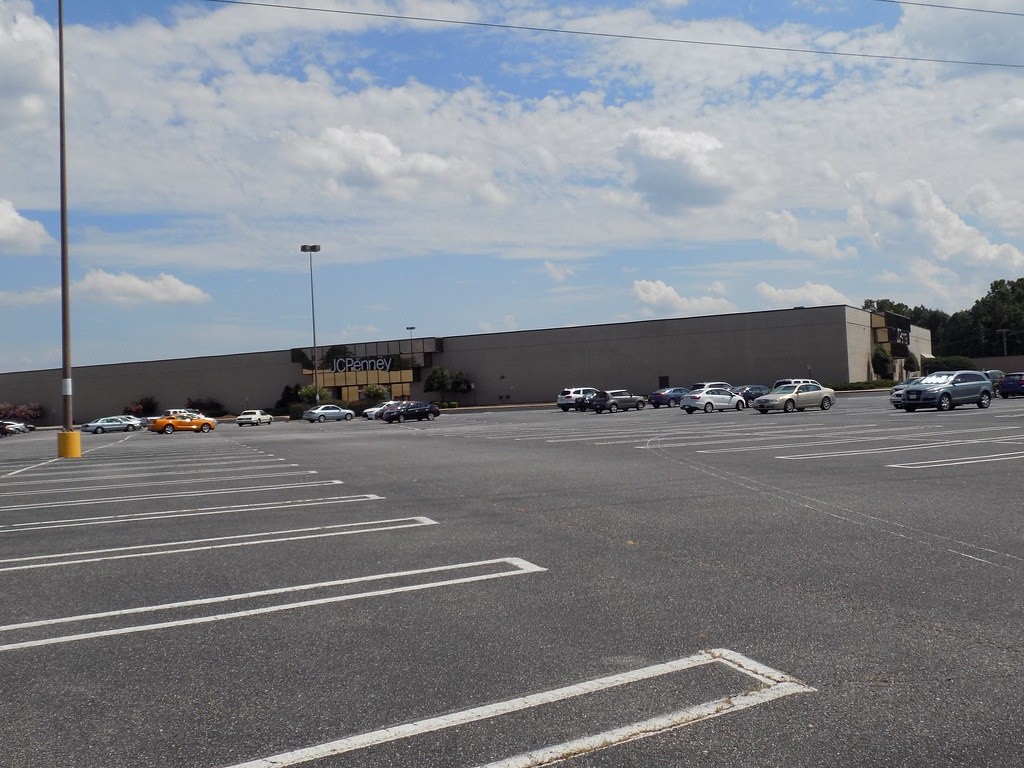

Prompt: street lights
[[405, 326, 417, 338], [300, 244, 321, 407]]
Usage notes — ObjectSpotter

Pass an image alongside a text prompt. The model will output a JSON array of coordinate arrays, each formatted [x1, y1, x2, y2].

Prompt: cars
[[236, 410, 274, 426], [80, 408, 217, 434], [0, 420, 36, 438], [557, 369, 1024, 414], [302, 404, 355, 423], [362, 400, 441, 423]]
